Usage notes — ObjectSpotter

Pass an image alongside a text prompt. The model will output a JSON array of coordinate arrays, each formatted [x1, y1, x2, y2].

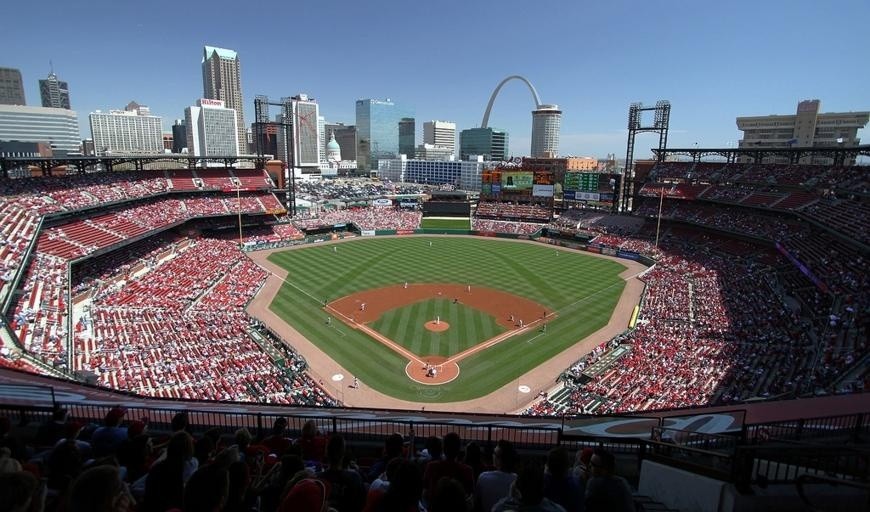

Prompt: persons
[[352, 279, 470, 389], [291, 172, 456, 241], [4, 162, 345, 407], [2, 407, 868, 510], [472, 162, 869, 417]]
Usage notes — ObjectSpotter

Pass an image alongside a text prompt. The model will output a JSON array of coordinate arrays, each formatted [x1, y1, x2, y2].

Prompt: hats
[[283, 476, 332, 512], [104, 406, 280, 464]]
